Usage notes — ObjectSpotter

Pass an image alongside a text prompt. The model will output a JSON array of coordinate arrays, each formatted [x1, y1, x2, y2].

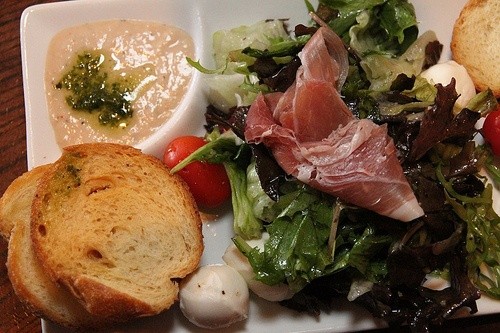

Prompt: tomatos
[[161, 135, 232, 209]]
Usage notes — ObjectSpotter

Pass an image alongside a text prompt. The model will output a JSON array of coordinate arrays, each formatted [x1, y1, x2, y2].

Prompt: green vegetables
[[168, 0, 500, 333], [0, 144, 204, 329]]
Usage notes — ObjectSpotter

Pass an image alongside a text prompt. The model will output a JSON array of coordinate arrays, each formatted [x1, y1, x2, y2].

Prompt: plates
[[19, 1, 499, 333]]
